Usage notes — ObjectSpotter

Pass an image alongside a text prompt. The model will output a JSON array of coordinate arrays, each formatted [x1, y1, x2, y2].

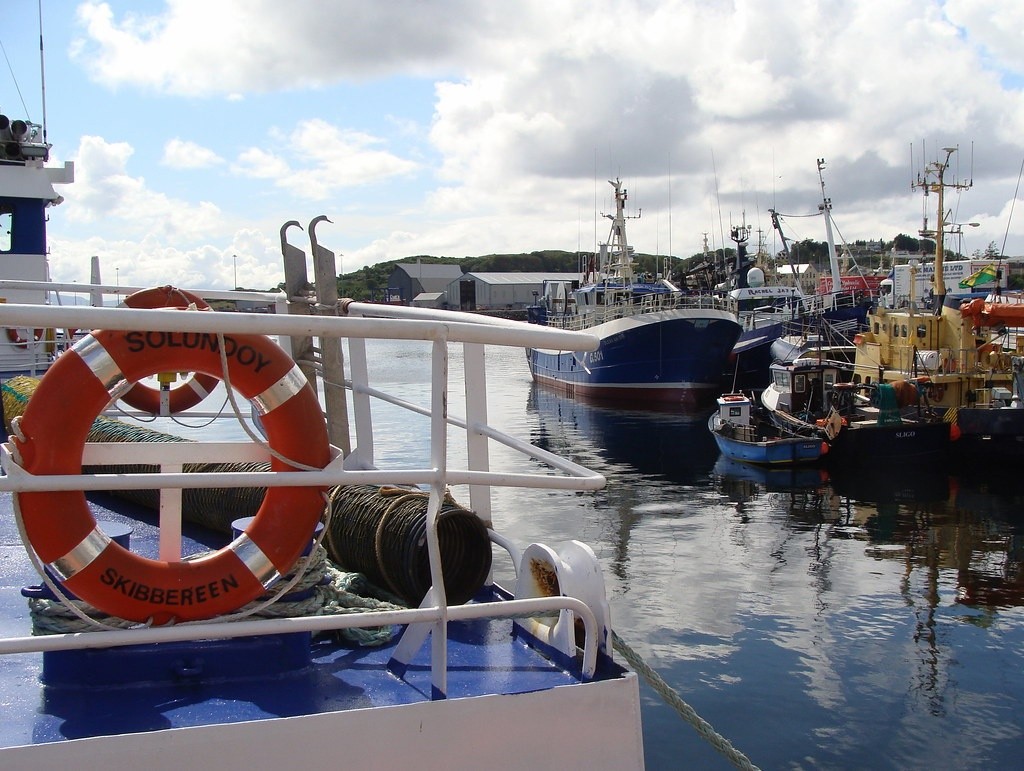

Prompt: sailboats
[[525, 138, 1024, 449]]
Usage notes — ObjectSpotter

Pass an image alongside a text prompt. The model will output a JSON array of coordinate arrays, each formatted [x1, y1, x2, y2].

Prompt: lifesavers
[[5, 288, 327, 625], [724, 395, 744, 402]]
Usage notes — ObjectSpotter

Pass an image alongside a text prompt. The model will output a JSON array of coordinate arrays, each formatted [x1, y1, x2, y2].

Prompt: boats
[[0, 112, 643, 771], [708, 403, 821, 463]]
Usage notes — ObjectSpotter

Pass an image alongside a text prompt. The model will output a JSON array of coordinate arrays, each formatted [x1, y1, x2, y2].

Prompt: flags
[[958, 263, 997, 289]]
